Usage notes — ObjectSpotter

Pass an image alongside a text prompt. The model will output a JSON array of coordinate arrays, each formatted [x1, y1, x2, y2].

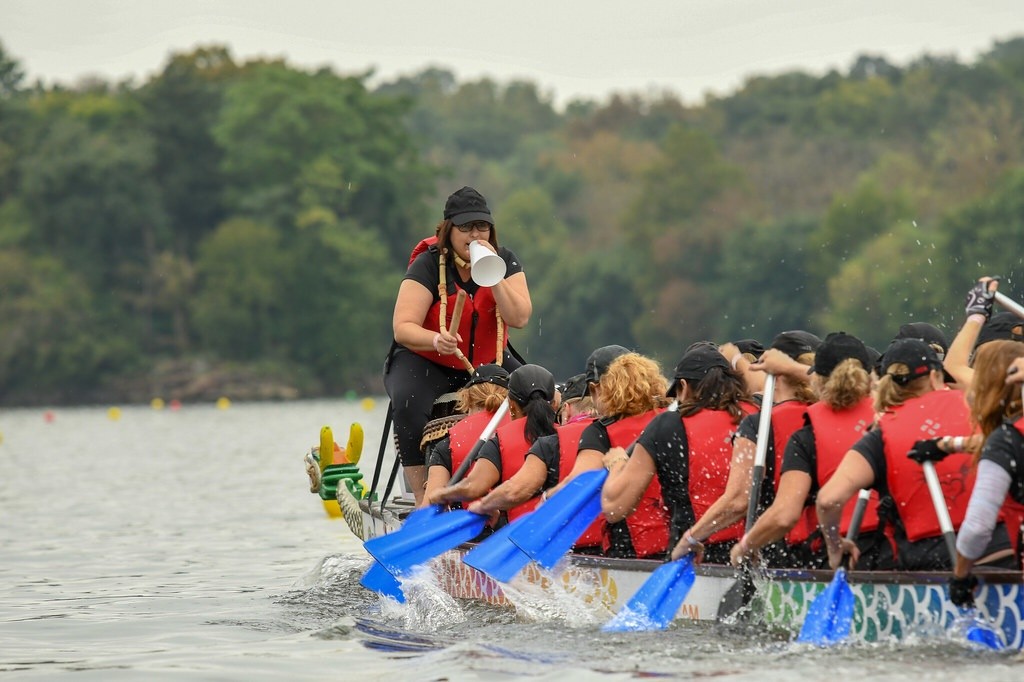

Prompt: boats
[[302, 423, 1024, 660]]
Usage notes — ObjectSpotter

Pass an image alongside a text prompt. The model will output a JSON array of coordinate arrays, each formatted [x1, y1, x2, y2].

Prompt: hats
[[580, 344, 632, 401], [444, 186, 494, 225], [665, 346, 732, 398], [805, 332, 873, 380], [882, 337, 944, 385], [508, 364, 555, 403], [866, 345, 881, 373], [876, 322, 948, 361], [969, 312, 1024, 368], [733, 339, 764, 363], [560, 373, 593, 403], [465, 364, 509, 389], [749, 331, 821, 364], [687, 341, 717, 356]]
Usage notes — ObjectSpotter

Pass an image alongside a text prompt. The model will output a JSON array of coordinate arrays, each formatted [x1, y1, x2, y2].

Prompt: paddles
[[506, 398, 681, 570], [992, 291, 1024, 320], [714, 372, 777, 639], [361, 486, 551, 582], [356, 395, 510, 605], [917, 454, 1006, 654], [598, 547, 699, 631], [794, 483, 871, 646], [460, 508, 532, 587]]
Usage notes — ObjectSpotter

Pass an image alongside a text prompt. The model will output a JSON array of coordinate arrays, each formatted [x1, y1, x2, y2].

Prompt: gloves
[[906, 437, 947, 465], [949, 572, 978, 609]]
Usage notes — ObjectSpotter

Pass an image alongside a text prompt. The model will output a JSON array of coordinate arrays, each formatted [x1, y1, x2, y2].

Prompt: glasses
[[453, 222, 491, 232]]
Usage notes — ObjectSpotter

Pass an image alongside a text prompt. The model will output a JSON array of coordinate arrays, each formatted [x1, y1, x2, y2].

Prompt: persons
[[417, 272, 1024, 606], [381, 186, 531, 508]]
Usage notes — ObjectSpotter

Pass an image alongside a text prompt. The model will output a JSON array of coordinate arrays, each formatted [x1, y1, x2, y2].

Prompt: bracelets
[[684, 530, 697, 544], [740, 535, 750, 552], [954, 436, 964, 451], [732, 354, 743, 370], [432, 334, 440, 351]]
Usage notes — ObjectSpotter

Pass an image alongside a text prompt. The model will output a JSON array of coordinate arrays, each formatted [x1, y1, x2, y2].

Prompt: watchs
[[943, 436, 955, 453]]
